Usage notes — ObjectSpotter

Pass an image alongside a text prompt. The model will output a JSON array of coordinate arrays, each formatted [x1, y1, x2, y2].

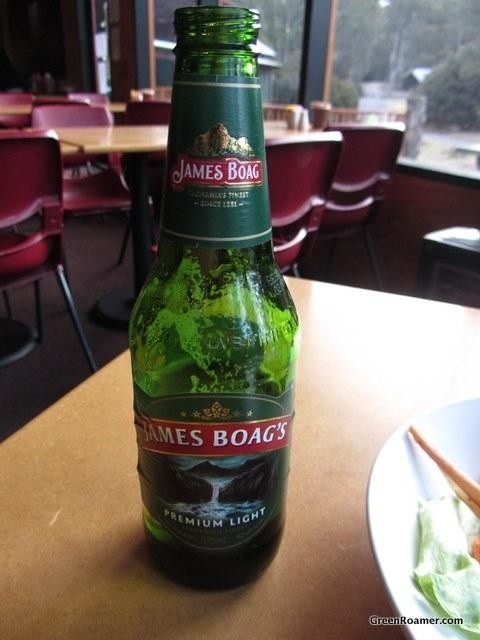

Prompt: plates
[[365, 396, 480, 635]]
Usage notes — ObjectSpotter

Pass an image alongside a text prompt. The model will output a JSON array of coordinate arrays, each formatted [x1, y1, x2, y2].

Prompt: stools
[[420, 223, 479, 307]]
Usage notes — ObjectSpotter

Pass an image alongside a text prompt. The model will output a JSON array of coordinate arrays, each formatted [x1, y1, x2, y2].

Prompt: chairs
[[0, 88, 410, 371]]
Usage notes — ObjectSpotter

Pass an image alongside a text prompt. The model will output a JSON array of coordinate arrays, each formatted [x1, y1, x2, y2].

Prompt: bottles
[[129, 6, 297, 592]]
